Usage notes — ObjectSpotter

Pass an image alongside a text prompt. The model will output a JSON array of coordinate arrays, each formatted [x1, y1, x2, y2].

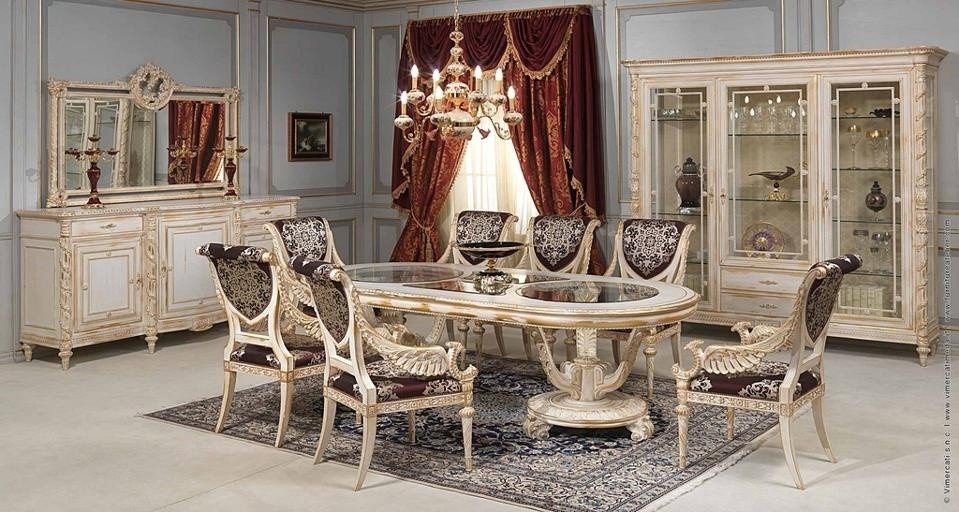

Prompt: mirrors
[[47, 63, 248, 208]]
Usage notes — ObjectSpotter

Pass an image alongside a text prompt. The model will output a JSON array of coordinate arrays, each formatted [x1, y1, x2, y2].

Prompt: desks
[[303, 261, 703, 446]]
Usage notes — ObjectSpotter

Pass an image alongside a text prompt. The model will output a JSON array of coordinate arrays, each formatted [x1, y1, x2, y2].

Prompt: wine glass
[[844, 122, 863, 170], [866, 127, 894, 169], [853, 230, 893, 273]]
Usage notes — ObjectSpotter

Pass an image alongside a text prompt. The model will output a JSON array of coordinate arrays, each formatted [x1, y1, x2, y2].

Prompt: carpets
[[139, 343, 819, 511]]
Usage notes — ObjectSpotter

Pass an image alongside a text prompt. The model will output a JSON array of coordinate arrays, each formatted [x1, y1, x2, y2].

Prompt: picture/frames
[[286, 110, 337, 162]]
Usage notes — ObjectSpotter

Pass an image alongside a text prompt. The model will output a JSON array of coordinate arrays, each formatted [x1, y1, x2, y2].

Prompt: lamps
[[167, 130, 205, 184], [64, 111, 124, 207], [390, 1, 526, 144], [212, 129, 253, 200], [723, 92, 808, 144]]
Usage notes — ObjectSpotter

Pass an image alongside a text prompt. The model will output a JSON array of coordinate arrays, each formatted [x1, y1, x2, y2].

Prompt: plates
[[743, 222, 785, 258]]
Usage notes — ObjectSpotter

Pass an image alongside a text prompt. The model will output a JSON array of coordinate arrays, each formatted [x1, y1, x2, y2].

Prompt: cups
[[737, 106, 808, 135]]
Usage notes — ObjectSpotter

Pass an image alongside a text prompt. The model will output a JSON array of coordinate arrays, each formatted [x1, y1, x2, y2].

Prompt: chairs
[[436, 211, 524, 355], [192, 241, 365, 449], [672, 246, 867, 491], [566, 218, 695, 399], [493, 213, 603, 364], [285, 252, 481, 492]]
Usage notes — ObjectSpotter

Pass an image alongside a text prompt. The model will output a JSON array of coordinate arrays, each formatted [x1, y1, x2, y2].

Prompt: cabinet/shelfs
[[16, 196, 299, 370], [619, 46, 950, 366]]
